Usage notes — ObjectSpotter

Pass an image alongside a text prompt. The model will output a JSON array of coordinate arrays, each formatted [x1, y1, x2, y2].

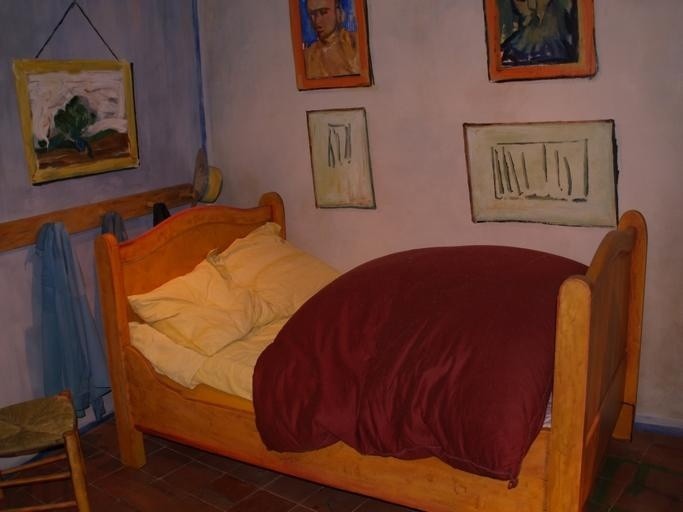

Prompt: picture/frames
[[287, 0, 374, 93], [461, 0, 623, 230], [11, 59, 140, 189], [304, 107, 376, 212]]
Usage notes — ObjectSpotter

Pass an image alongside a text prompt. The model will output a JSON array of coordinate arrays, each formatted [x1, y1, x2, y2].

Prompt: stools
[[2, 387, 91, 512]]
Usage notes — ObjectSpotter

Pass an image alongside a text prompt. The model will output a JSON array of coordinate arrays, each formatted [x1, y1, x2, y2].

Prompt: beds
[[96, 189, 649, 511]]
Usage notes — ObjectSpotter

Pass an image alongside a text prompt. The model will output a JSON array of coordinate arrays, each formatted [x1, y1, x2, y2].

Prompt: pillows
[[127, 220, 345, 359]]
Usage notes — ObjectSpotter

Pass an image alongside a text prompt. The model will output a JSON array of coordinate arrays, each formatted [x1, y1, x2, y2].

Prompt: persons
[[303, 0, 358, 78]]
